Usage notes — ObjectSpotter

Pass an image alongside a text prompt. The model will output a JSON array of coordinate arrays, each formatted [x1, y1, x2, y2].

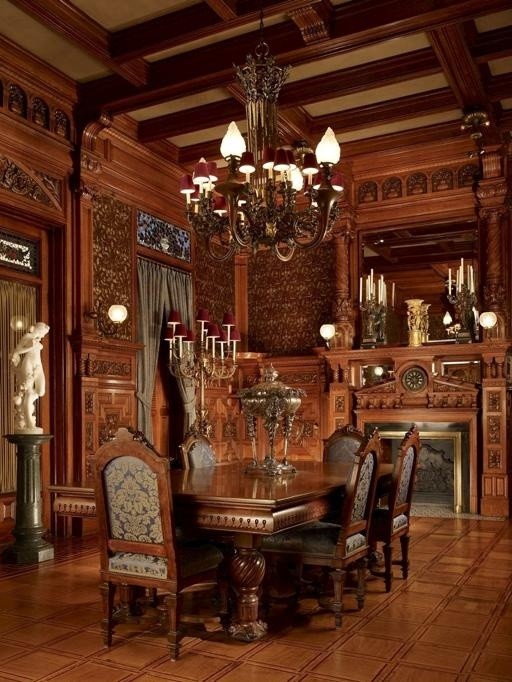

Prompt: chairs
[[178, 426, 217, 468], [369, 426, 422, 590], [93, 425, 233, 660], [318, 426, 366, 471], [262, 426, 385, 631]]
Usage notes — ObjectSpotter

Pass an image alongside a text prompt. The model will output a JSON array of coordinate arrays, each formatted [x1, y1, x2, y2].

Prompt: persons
[[11, 322, 51, 429]]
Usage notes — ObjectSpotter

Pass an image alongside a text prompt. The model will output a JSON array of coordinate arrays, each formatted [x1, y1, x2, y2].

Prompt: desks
[[49, 451, 348, 645]]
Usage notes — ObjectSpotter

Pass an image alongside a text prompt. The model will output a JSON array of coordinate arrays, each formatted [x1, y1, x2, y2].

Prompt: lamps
[[178, 0, 347, 263], [320, 323, 335, 346], [107, 301, 127, 340], [479, 310, 497, 339]]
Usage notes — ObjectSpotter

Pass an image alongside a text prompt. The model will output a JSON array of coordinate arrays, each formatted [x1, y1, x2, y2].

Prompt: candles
[[358, 268, 396, 308], [447, 256, 476, 294]]
